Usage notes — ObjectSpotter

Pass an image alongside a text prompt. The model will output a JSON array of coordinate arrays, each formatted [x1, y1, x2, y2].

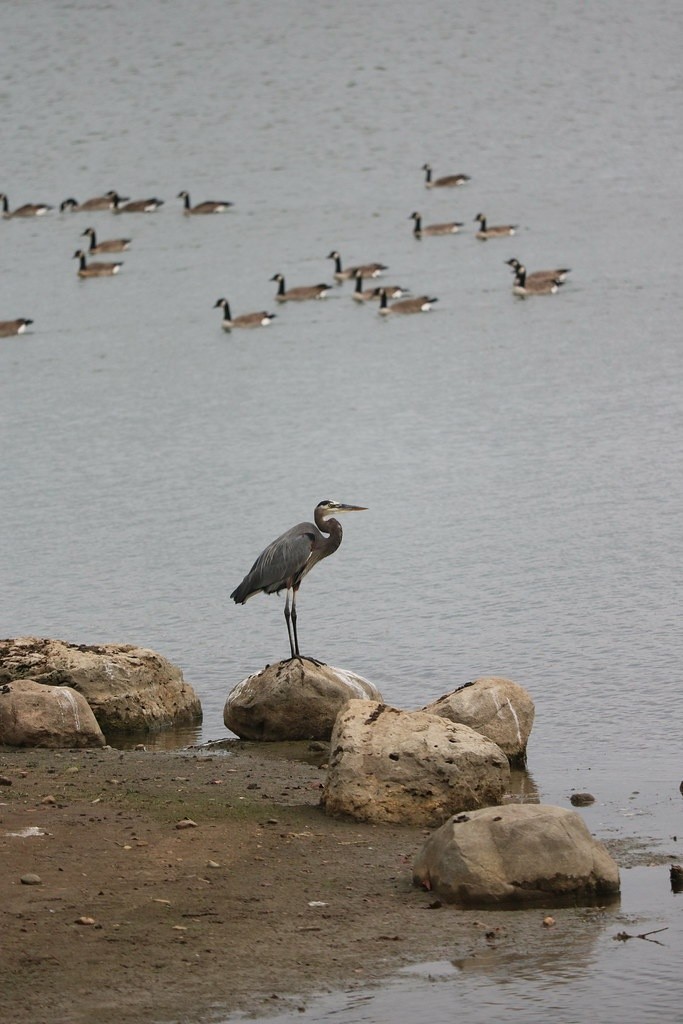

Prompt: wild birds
[[228, 499, 371, 669], [0, 163, 572, 339]]
